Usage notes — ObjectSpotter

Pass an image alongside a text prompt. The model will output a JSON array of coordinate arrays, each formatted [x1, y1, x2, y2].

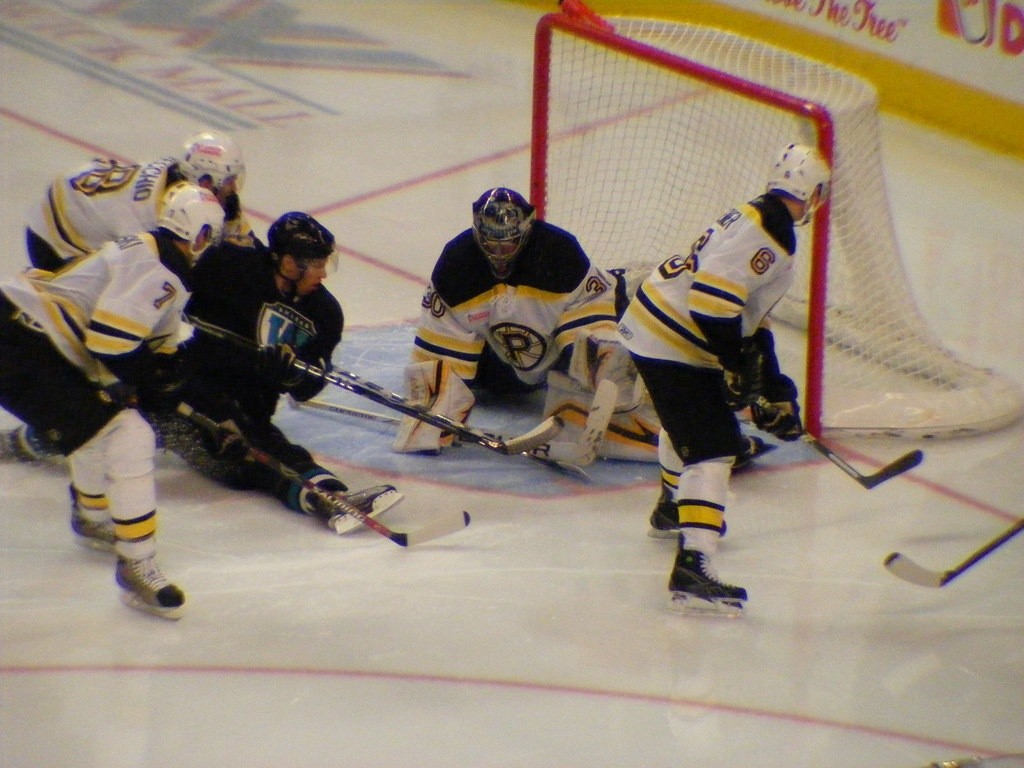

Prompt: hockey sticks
[[175, 399, 475, 550], [179, 313, 567, 458], [330, 363, 595, 487], [881, 518, 1024, 590], [754, 394, 926, 491], [288, 378, 621, 468]]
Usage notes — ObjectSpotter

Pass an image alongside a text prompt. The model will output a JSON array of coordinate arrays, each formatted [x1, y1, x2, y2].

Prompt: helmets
[[267, 212, 333, 260], [155, 179, 225, 248], [766, 142, 831, 207], [472, 186, 535, 275], [176, 131, 242, 193]]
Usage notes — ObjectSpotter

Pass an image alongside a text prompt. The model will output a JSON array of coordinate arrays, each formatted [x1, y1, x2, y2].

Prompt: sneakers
[[668, 532, 748, 618], [69, 483, 118, 553], [114, 553, 184, 620], [648, 484, 725, 539], [0, 422, 42, 462]]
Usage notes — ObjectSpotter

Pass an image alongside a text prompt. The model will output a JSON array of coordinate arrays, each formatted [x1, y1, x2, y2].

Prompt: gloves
[[750, 374, 803, 442], [133, 345, 187, 425], [721, 327, 779, 412], [263, 343, 299, 386]]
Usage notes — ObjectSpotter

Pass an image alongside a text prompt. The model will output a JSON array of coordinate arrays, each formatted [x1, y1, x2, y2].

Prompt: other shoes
[[318, 483, 405, 536]]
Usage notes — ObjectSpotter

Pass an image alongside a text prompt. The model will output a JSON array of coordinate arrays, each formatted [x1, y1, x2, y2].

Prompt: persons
[[0, 209, 398, 530], [392, 142, 831, 604], [0, 181, 226, 608], [25, 129, 269, 269]]
